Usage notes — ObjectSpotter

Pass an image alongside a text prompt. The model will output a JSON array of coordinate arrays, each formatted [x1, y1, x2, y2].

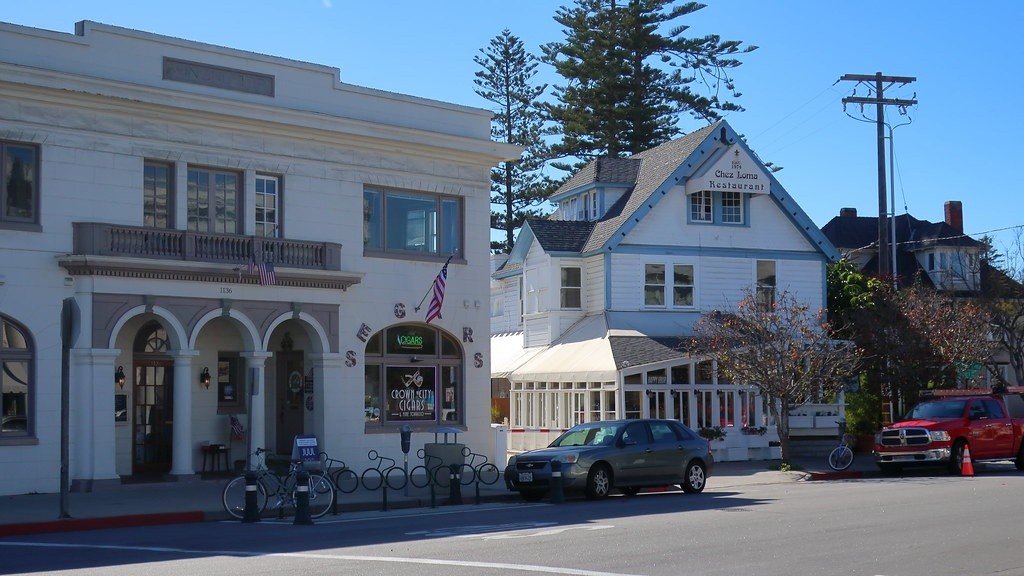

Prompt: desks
[[200, 442, 231, 480]]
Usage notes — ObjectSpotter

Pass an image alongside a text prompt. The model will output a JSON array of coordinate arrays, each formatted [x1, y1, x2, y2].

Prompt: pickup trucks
[[872, 396, 1024, 473]]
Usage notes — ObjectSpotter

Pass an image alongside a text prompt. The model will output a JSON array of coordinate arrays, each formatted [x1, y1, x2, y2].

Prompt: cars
[[0, 416, 27, 430], [503, 419, 714, 501]]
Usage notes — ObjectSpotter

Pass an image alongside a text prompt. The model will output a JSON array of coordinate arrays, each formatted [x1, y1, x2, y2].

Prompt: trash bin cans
[[424, 427, 466, 494]]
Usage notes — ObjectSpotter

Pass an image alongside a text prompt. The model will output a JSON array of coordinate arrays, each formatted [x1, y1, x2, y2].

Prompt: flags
[[258, 262, 275, 286], [425, 258, 451, 324], [231, 416, 241, 435]]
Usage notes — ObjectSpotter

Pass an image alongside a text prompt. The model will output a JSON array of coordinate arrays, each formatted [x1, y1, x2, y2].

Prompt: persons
[[447, 392, 453, 401]]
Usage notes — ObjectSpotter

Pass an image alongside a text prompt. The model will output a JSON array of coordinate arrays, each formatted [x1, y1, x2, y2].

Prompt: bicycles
[[222, 447, 336, 519], [829, 420, 854, 471]]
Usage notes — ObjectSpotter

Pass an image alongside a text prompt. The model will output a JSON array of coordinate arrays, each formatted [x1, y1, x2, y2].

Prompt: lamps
[[113, 367, 129, 388], [199, 366, 212, 390]]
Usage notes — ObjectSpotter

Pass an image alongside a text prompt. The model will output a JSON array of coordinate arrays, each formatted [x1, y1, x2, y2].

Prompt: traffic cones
[[960, 444, 976, 476]]
